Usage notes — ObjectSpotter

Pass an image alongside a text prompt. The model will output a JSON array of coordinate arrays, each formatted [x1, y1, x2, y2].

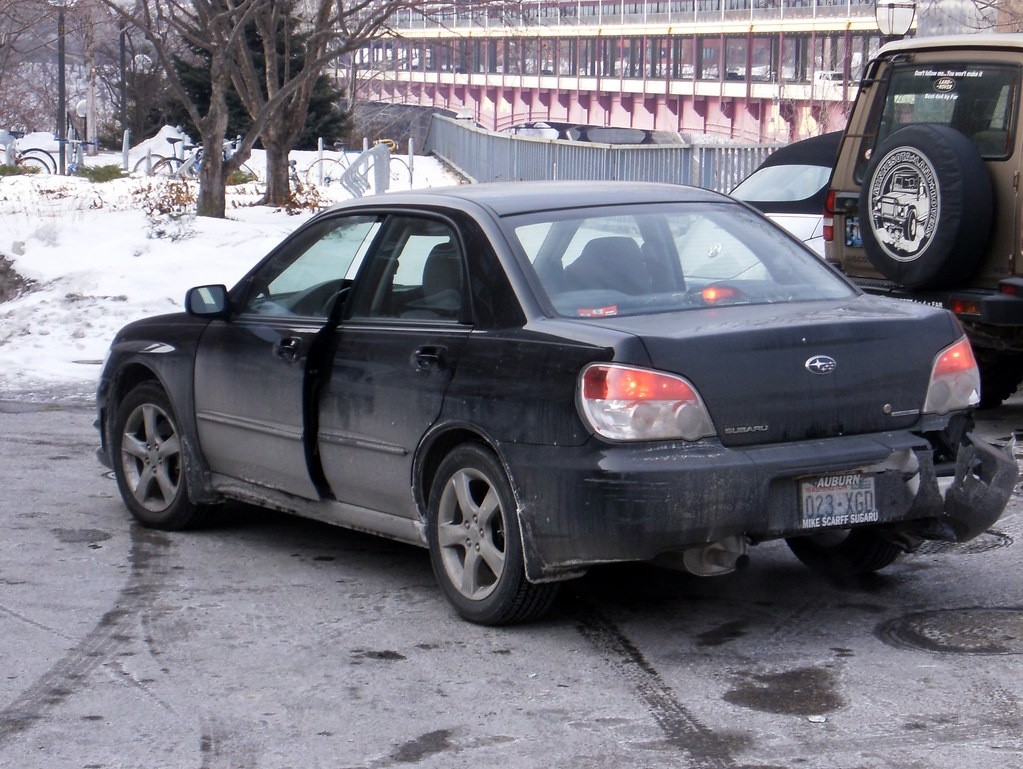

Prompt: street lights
[[47, 0, 77, 179], [131, 54, 152, 145]]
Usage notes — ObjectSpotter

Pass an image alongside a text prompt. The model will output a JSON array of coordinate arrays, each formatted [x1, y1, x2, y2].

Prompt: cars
[[817, 72, 855, 87], [663, 131, 848, 298], [93, 179, 1014, 627]]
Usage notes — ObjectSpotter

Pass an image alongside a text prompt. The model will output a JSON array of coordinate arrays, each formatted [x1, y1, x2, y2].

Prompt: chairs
[[969, 130, 1006, 157], [564, 234, 645, 292], [421, 240, 461, 311]]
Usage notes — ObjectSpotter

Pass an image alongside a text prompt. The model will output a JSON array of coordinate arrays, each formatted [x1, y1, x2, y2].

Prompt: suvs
[[820, 33, 1022, 415]]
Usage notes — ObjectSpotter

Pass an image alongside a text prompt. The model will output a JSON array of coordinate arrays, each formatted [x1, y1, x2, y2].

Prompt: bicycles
[[304, 142, 414, 194], [0, 130, 59, 177], [132, 137, 242, 183]]
